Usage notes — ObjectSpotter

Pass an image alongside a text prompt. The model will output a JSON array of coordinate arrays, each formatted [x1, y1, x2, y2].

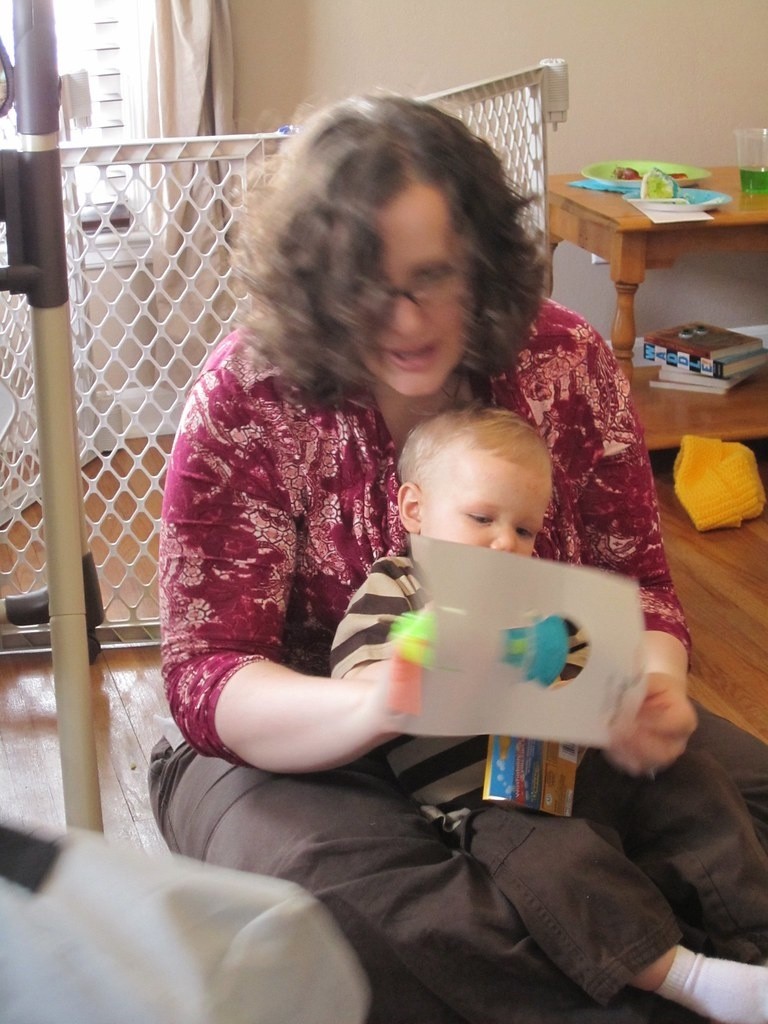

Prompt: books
[[644, 322, 768, 395]]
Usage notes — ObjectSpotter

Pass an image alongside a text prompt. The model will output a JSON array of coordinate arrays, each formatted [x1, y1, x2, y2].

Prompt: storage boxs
[[481, 735, 577, 817]]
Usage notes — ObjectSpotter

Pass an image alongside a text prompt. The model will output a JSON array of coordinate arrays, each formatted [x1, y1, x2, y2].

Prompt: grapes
[[616, 167, 688, 181]]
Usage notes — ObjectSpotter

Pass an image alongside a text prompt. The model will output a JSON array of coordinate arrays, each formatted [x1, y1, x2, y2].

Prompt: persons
[[328, 409, 768, 1024], [149, 94, 768, 1023]]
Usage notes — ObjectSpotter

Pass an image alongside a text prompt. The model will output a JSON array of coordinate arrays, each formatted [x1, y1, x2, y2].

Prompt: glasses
[[345, 265, 476, 323]]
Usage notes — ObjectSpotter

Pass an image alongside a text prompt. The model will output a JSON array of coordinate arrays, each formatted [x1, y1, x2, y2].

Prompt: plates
[[622, 189, 732, 212], [580, 159, 712, 188]]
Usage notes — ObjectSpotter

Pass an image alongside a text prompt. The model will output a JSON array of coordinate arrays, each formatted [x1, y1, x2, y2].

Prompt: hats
[[673, 435, 767, 532]]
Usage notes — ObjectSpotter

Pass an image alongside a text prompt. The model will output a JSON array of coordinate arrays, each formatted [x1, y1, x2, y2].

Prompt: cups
[[734, 128, 768, 194]]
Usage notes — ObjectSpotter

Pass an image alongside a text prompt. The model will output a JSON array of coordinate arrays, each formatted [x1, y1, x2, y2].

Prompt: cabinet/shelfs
[[547, 166, 768, 449]]
